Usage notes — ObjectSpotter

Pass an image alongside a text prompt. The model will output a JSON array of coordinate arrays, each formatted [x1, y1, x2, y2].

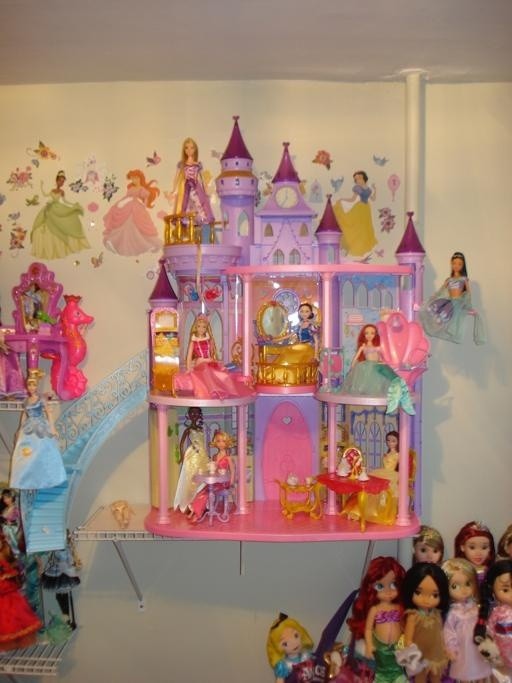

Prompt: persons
[[351, 324, 417, 416], [422, 257, 471, 343], [173, 408, 235, 520], [145, 114, 512, 683], [171, 138, 215, 245], [266, 522, 512, 683], [264, 304, 319, 383], [177, 314, 252, 399], [342, 429, 399, 525]]
